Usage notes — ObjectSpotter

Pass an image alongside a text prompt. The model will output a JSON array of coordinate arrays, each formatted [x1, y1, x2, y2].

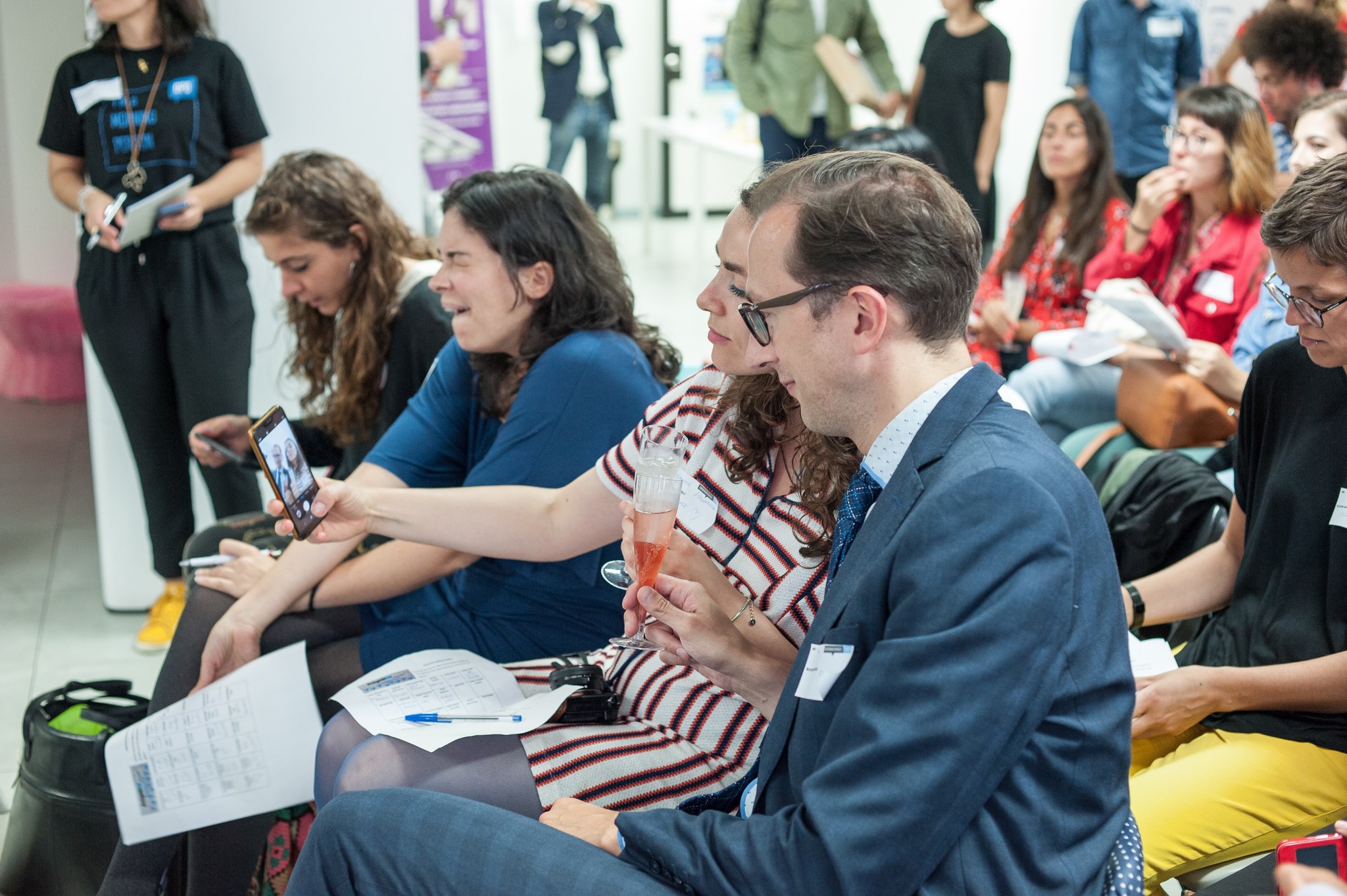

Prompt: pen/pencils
[[405, 712, 522, 723], [179, 549, 282, 568], [86, 192, 127, 250], [159, 201, 189, 214]]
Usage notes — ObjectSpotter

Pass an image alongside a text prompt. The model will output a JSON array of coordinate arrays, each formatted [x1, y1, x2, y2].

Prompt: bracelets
[[1126, 218, 1152, 235], [309, 580, 318, 611], [731, 596, 756, 626], [77, 184, 93, 212], [1121, 581, 1145, 632]]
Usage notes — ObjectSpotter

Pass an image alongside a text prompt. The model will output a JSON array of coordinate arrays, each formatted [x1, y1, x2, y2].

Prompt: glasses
[[737, 282, 835, 347], [1163, 125, 1207, 158], [1262, 271, 1347, 328]]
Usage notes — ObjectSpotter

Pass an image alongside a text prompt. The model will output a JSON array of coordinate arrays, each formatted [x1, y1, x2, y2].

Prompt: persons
[[283, 148, 1145, 894], [270, 150, 864, 824], [1273, 819, 1347, 895], [180, 152, 448, 598], [723, 0, 902, 177], [1198, 1, 1347, 126], [98, 163, 681, 896], [1057, 88, 1347, 505], [270, 438, 313, 507], [964, 94, 1129, 383], [1065, 0, 1202, 207], [1231, 6, 1347, 192], [904, 0, 1011, 272], [1005, 81, 1294, 447], [537, 0, 624, 217], [1122, 151, 1347, 896], [420, 32, 465, 75], [38, 1, 271, 655]]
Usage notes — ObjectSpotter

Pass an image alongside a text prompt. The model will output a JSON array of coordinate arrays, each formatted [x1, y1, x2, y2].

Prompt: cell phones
[[152, 201, 189, 237], [194, 433, 262, 470], [248, 405, 329, 542]]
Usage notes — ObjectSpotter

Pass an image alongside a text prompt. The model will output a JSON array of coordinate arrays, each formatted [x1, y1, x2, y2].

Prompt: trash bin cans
[[2, 284, 86, 400]]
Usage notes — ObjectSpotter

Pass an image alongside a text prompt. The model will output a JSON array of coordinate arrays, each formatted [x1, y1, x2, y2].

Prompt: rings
[[91, 223, 100, 234]]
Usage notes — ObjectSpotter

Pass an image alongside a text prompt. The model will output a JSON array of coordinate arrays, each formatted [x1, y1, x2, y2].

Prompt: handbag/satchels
[[0, 680, 153, 896], [1114, 356, 1242, 450]]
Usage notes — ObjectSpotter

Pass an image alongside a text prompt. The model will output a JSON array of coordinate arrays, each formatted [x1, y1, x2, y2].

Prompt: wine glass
[[601, 423, 689, 591], [608, 473, 684, 652]]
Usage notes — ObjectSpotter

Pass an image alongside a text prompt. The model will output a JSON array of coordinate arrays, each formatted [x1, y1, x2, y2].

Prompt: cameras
[[1276, 832, 1347, 896]]
